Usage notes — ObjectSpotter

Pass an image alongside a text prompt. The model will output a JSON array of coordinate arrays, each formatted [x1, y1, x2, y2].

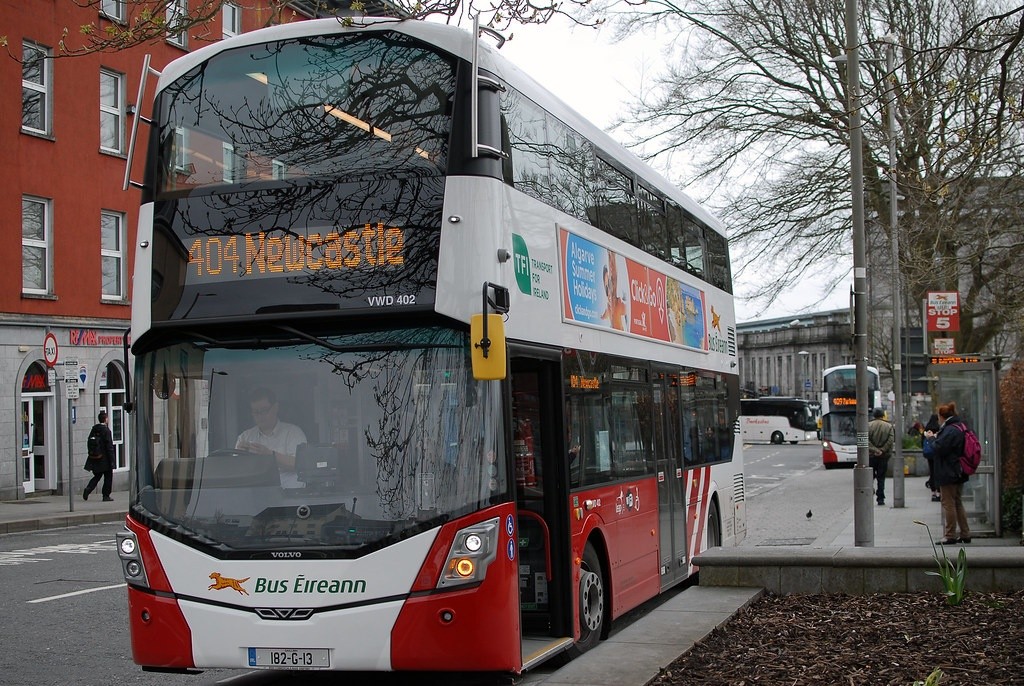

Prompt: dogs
[[207, 572, 251, 597], [710, 305, 722, 333]]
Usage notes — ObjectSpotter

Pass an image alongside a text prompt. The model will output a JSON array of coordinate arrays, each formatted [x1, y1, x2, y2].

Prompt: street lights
[[798, 351, 809, 400], [829, 42, 913, 508]]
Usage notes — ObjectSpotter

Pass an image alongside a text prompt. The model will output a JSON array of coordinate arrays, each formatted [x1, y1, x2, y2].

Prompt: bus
[[115, 14, 748, 677], [815, 364, 882, 469], [740, 396, 817, 446]]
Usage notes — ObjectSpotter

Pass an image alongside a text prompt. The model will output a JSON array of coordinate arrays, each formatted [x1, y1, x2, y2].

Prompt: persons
[[926, 402, 972, 546], [922, 413, 943, 501], [81, 413, 116, 501], [867, 407, 895, 505], [682, 420, 734, 467], [229, 385, 309, 491], [599, 247, 628, 331]]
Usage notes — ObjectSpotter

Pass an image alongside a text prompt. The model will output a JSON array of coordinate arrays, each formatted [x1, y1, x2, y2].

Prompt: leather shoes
[[935, 537, 971, 544]]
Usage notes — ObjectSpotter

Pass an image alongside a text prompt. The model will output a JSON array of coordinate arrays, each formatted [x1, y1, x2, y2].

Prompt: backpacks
[[88, 427, 109, 459], [923, 437, 937, 459], [953, 423, 982, 475]]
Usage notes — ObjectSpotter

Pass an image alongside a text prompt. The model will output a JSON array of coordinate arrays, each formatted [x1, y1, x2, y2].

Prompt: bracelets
[[271, 450, 276, 456]]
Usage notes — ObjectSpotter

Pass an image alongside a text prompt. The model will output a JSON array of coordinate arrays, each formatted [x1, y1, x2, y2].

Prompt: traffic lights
[[348, 529, 355, 534]]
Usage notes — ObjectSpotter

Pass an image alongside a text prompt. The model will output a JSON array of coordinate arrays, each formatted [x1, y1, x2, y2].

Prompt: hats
[[872, 408, 886, 416]]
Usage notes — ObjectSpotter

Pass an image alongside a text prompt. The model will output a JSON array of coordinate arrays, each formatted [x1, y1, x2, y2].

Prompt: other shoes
[[931, 491, 941, 501], [876, 499, 885, 505], [102, 495, 115, 501], [82, 488, 90, 502]]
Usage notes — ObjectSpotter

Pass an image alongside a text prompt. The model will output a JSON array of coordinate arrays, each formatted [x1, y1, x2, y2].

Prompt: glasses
[[250, 404, 274, 416]]
